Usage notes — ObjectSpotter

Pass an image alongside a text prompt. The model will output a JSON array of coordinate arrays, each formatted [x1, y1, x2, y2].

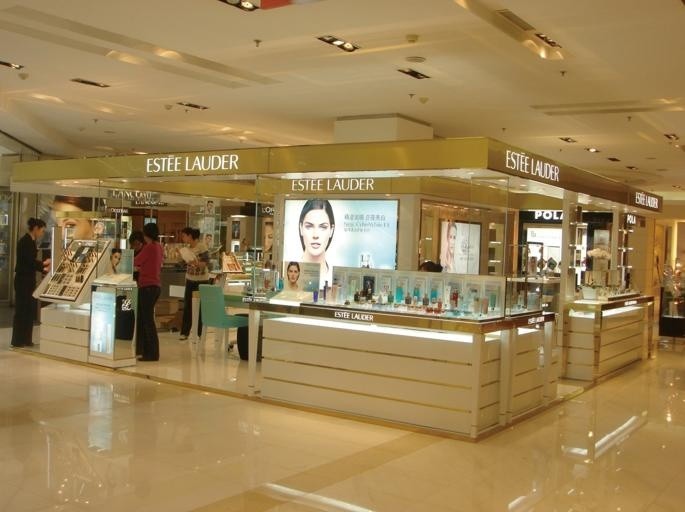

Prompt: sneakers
[[139, 357, 158, 361], [178, 334, 187, 339]]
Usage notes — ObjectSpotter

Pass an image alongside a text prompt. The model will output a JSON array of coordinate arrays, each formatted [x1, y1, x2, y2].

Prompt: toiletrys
[[482, 296, 489, 315], [407, 292, 412, 304], [319, 290, 323, 299], [438, 295, 442, 308], [413, 296, 417, 305], [396, 281, 400, 302], [431, 287, 437, 304], [453, 288, 458, 307], [313, 290, 318, 302], [328, 287, 331, 303], [323, 281, 328, 303], [333, 283, 337, 303], [423, 293, 429, 305], [360, 254, 370, 268], [387, 291, 394, 304], [489, 290, 497, 311], [474, 296, 480, 314], [354, 291, 360, 301], [336, 285, 343, 304], [414, 284, 421, 302], [404, 297, 408, 305], [366, 289, 372, 301]]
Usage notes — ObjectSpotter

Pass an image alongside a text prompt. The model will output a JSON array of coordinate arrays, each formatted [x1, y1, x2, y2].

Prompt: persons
[[128, 231, 145, 280], [205, 234, 212, 248], [265, 221, 274, 252], [134, 221, 163, 362], [285, 262, 301, 289], [420, 262, 444, 272], [297, 200, 335, 273], [177, 227, 212, 341], [107, 249, 121, 274], [11, 218, 53, 349], [443, 222, 457, 272], [205, 201, 213, 217], [50, 193, 104, 246]]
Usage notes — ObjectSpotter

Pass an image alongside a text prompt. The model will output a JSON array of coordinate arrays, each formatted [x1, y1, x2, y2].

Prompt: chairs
[[199, 284, 248, 367]]
